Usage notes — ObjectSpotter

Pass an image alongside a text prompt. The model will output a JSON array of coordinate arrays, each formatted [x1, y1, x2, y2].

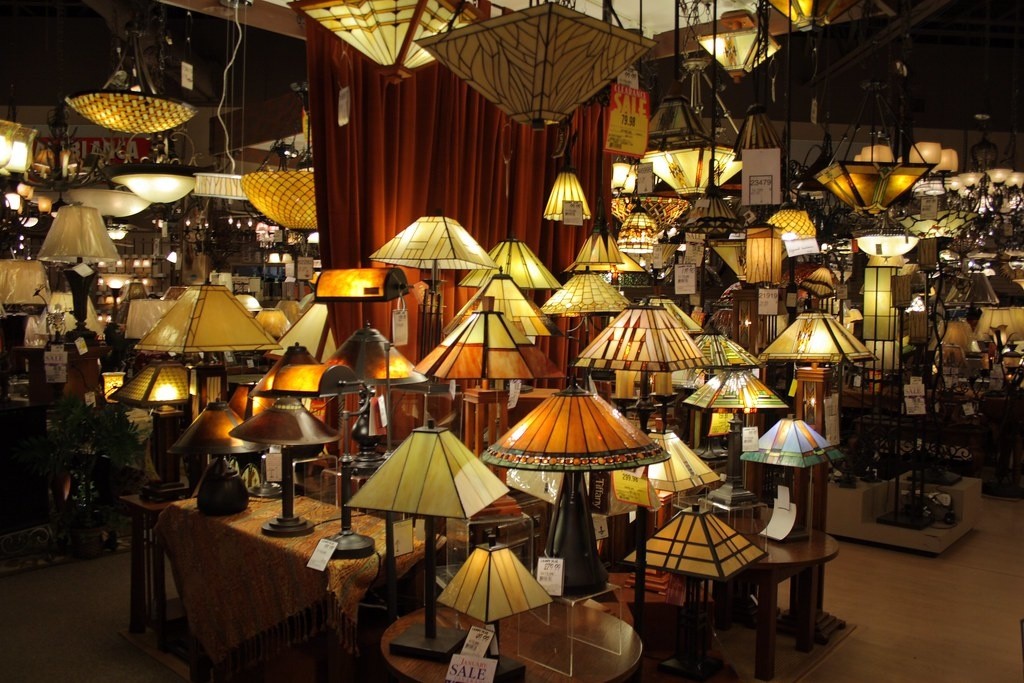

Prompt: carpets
[[119, 611, 858, 682]]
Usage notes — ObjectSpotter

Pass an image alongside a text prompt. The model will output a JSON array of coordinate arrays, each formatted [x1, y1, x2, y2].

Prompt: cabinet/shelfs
[[0, 404, 64, 578]]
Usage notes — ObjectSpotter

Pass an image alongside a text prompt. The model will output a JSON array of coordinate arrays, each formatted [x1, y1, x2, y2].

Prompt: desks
[[692, 518, 839, 681], [382, 606, 643, 683], [120, 495, 447, 683]]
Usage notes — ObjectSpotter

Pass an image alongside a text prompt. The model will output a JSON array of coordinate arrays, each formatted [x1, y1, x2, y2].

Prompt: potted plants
[[12, 404, 143, 559]]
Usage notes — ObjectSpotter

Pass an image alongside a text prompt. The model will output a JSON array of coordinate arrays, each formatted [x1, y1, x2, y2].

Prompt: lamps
[[1, 0, 1024, 683]]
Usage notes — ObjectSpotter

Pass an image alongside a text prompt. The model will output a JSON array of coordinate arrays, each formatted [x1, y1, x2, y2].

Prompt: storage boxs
[[826, 471, 980, 554]]
